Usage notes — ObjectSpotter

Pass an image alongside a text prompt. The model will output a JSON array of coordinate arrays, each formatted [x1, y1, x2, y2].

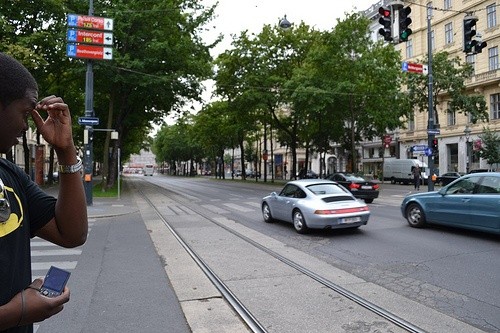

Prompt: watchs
[[57, 156, 83, 173]]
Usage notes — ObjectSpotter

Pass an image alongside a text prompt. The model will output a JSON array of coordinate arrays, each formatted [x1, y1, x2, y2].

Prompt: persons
[[412, 165, 422, 190], [0, 55, 89, 333]]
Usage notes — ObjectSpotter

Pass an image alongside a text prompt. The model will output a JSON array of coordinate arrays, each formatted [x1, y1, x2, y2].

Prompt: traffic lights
[[474, 34, 483, 53], [398, 5, 412, 43], [66, 43, 113, 60], [433, 137, 440, 154], [377, 4, 395, 42], [462, 18, 479, 53]]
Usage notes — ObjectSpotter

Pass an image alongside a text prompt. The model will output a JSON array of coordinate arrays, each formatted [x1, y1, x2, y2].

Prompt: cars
[[235, 169, 260, 178], [306, 170, 319, 179], [326, 170, 379, 204], [399, 171, 500, 233], [436, 171, 468, 187], [259, 177, 371, 235]]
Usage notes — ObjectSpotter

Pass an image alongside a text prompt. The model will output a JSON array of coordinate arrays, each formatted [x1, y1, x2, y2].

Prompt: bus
[[143, 164, 154, 176]]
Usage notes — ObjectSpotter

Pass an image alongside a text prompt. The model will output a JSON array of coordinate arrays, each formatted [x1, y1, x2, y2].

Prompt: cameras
[[39, 265, 71, 297]]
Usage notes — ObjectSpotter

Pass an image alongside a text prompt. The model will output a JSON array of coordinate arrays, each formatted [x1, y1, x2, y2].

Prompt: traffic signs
[[411, 145, 433, 157], [67, 14, 114, 31], [402, 61, 428, 75], [66, 28, 113, 46]]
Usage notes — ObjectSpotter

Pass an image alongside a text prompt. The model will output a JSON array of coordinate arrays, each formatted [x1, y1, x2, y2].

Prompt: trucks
[[383, 157, 437, 184]]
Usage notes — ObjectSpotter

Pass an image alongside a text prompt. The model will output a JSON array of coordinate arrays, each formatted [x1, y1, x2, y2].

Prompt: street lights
[[463, 124, 472, 173], [279, 12, 292, 29]]
